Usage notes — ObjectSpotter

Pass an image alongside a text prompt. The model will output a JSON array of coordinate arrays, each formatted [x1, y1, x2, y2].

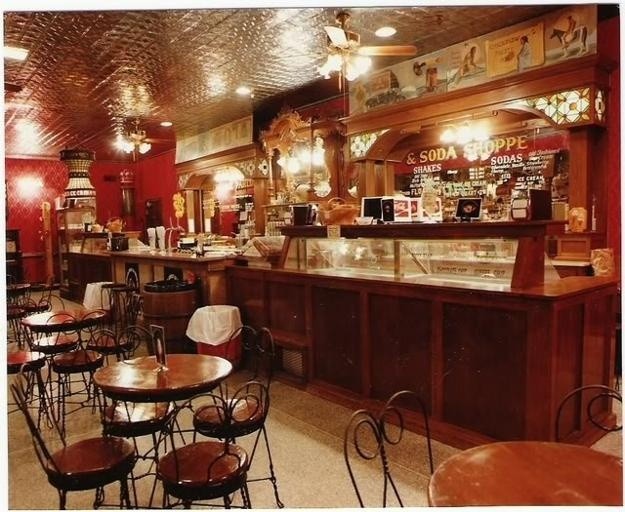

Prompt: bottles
[[590, 195, 601, 231], [265, 221, 284, 236]]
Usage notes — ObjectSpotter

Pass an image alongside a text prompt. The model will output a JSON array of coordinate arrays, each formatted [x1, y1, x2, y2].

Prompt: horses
[[548, 25, 588, 57]]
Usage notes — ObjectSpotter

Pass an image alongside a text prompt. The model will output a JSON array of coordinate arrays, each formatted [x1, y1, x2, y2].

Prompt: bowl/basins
[[355, 216, 373, 226]]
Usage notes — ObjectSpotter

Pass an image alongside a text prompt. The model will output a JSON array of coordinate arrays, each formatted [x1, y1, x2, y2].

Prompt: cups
[[292, 206, 316, 226], [154, 226, 166, 251], [146, 228, 157, 250], [84, 223, 92, 232]]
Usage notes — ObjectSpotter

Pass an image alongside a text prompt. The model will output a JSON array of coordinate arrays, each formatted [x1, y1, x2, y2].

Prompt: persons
[[459, 46, 481, 75], [561, 16, 575, 49], [517, 35, 533, 72]]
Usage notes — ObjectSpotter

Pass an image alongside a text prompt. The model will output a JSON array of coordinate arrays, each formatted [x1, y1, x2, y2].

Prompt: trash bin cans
[[142, 280, 200, 356], [196, 305, 243, 370], [87, 281, 114, 324]]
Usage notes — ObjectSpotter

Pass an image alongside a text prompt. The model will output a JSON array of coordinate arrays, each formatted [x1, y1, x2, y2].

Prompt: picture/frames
[[361, 195, 482, 225]]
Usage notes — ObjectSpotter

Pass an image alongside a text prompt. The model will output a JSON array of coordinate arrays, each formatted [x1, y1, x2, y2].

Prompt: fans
[[323, 12, 418, 57]]
